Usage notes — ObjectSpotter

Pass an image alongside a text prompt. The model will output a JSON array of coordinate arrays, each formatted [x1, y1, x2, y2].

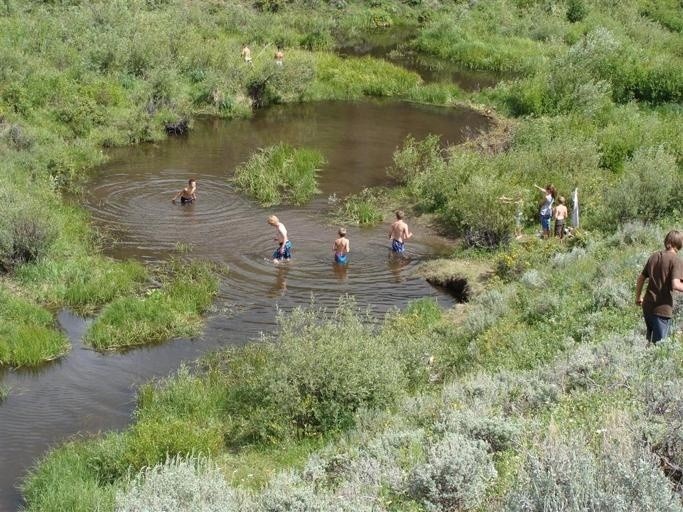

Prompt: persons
[[265, 264, 291, 301], [635, 230, 682, 348], [332, 228, 352, 264], [240, 42, 251, 63], [387, 210, 412, 252], [551, 197, 568, 239], [388, 252, 407, 284], [266, 215, 291, 264], [172, 178, 197, 205], [274, 45, 283, 66], [534, 183, 558, 240], [332, 263, 352, 293]]
